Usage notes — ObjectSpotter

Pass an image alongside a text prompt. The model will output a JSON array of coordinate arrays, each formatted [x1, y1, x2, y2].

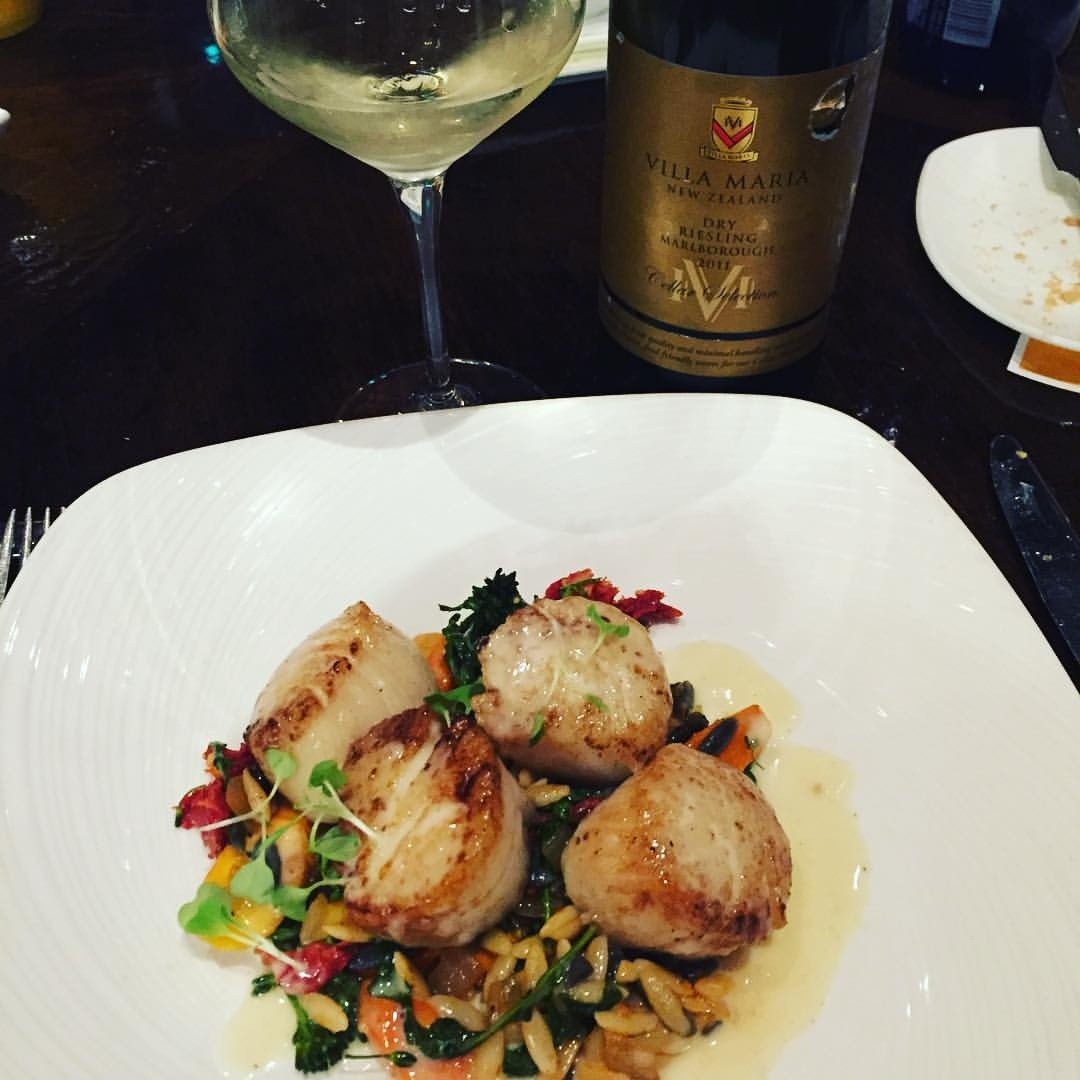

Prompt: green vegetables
[[178, 569, 766, 1076]]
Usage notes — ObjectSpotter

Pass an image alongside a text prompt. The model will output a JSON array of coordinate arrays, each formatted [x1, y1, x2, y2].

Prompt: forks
[[0, 504, 66, 602]]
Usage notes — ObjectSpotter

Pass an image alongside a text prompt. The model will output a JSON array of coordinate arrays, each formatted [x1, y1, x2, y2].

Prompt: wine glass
[[205, 1, 587, 423]]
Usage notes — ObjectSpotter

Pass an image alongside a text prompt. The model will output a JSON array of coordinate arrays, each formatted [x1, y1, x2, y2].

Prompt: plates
[[915, 124, 1079, 353], [0, 395, 1078, 1080]]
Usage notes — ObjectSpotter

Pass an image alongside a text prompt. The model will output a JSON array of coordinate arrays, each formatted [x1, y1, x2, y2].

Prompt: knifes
[[988, 434, 1080, 668]]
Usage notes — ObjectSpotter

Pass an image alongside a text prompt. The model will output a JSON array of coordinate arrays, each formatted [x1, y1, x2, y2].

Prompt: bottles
[[901, 0, 1023, 87], [590, 0, 891, 395]]
